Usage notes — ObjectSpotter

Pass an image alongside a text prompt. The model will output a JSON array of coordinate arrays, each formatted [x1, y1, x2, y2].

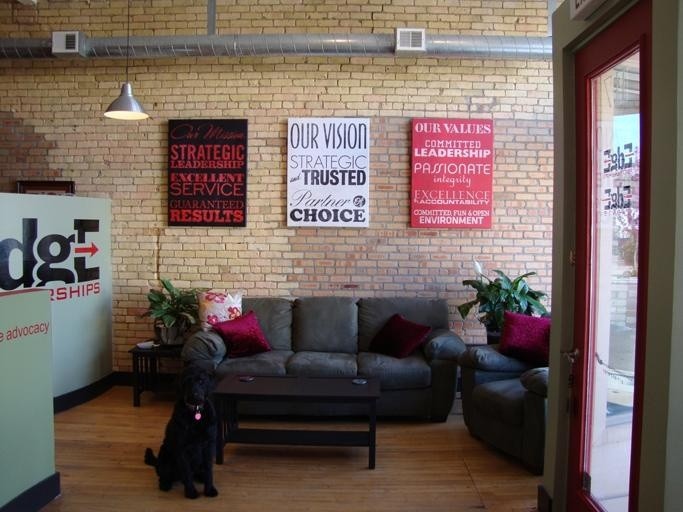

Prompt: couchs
[[180, 295, 466, 425]]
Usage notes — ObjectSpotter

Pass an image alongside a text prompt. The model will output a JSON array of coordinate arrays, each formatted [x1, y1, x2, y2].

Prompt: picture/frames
[[16, 179, 75, 196]]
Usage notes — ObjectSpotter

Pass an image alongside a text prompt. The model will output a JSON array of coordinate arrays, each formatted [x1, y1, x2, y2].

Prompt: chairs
[[460, 313, 550, 473]]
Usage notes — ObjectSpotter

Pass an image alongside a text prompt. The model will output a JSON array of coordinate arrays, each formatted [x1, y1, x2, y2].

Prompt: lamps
[[101, 0, 150, 121]]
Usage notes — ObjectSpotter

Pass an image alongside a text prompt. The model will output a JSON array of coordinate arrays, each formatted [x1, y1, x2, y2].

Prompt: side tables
[[129, 338, 184, 409]]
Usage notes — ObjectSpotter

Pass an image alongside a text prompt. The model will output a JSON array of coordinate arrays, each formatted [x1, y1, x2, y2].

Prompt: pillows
[[198, 292, 271, 359], [497, 309, 548, 362], [368, 314, 434, 357]]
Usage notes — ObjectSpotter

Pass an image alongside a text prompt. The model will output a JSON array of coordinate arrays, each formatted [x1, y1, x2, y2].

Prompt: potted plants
[[461, 257, 549, 343], [142, 276, 198, 348]]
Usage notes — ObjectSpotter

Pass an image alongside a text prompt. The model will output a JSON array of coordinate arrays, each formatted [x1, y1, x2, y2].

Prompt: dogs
[[143, 365, 219, 499]]
